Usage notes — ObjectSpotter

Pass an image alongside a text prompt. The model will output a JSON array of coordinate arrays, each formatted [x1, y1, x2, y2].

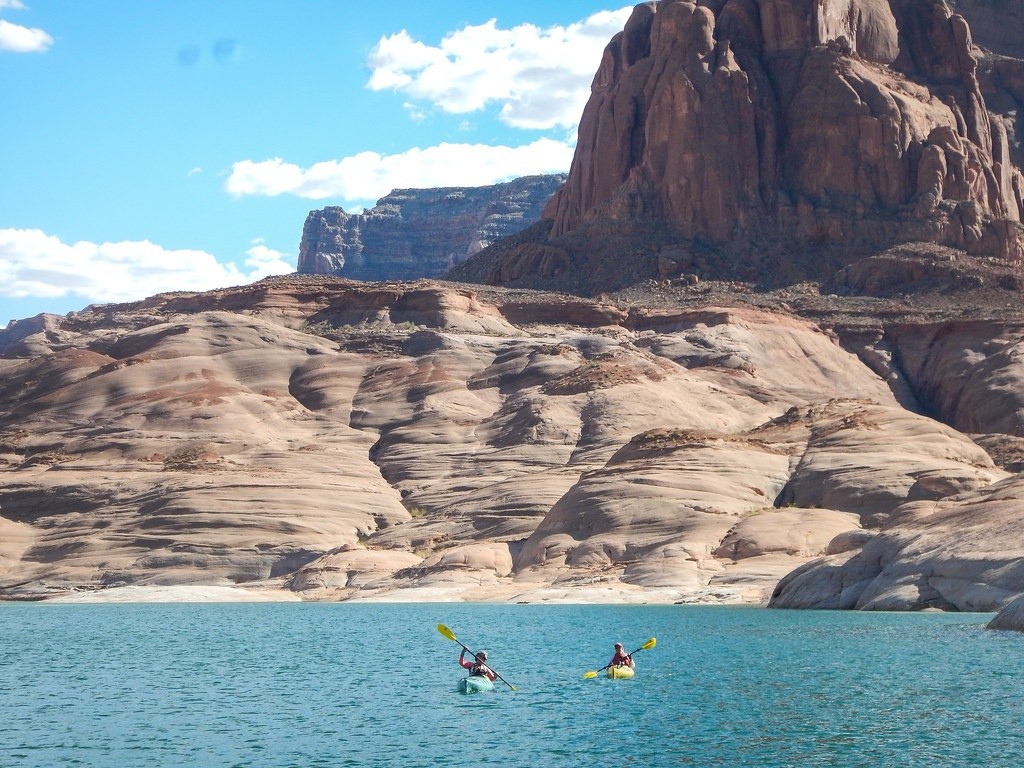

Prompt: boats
[[457, 674, 496, 695], [606, 665, 635, 681]]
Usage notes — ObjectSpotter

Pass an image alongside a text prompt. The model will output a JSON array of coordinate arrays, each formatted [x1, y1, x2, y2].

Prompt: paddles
[[583, 637, 656, 679], [436, 623, 518, 691]]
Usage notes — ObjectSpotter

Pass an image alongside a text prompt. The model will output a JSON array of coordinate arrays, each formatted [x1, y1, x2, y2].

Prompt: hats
[[476, 651, 488, 661], [614, 644, 623, 646]]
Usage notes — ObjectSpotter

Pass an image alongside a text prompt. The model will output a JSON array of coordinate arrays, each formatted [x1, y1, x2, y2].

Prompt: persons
[[459, 647, 499, 681], [609, 643, 635, 669]]
